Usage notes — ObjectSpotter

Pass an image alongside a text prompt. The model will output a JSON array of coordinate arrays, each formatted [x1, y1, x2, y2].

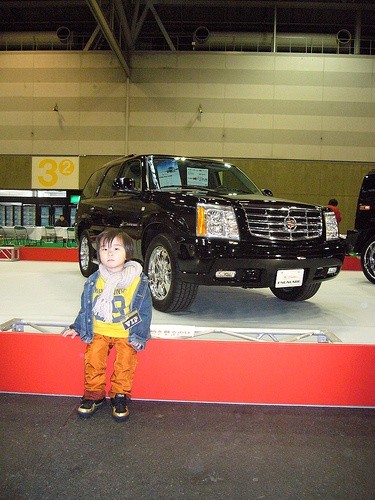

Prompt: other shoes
[[110, 397, 132, 420], [76, 395, 106, 417]]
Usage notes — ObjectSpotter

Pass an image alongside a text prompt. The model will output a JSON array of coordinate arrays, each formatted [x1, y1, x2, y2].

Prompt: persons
[[61, 228, 153, 420], [56, 216, 69, 228], [327, 199, 341, 223]]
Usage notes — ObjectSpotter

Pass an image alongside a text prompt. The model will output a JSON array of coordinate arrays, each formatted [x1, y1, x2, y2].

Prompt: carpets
[[341, 255, 363, 271]]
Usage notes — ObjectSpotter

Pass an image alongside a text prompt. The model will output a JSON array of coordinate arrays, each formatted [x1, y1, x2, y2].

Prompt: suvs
[[74, 153, 351, 314], [348, 170, 375, 284]]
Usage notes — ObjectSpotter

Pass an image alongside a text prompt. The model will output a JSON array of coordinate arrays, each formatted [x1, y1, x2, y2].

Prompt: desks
[[0, 226, 75, 249]]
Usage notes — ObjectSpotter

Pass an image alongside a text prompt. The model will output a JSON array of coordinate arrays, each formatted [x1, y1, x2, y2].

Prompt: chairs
[[14, 226, 30, 248], [0, 226, 8, 246], [63, 228, 78, 248], [45, 227, 57, 244]]
